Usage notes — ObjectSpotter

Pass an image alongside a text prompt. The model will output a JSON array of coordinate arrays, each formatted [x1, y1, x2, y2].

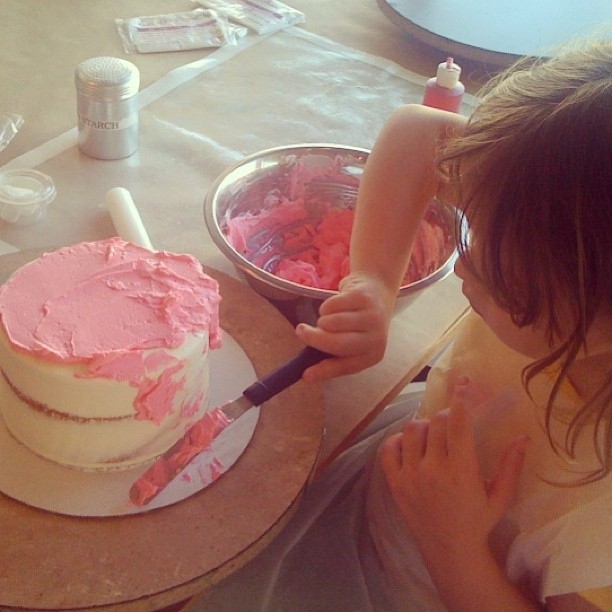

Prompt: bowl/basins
[[202, 143, 469, 330]]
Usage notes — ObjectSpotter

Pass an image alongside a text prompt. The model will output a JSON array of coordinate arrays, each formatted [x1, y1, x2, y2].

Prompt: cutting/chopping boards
[[1, 242, 324, 611]]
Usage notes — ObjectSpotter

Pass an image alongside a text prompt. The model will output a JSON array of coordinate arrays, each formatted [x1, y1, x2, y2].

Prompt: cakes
[[0, 234, 224, 472]]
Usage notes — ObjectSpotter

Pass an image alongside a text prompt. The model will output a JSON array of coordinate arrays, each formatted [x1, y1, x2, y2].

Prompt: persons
[[190, 37, 611, 611]]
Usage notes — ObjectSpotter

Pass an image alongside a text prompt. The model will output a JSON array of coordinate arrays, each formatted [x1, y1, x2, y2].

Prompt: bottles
[[421, 56, 466, 115], [75, 56, 140, 160]]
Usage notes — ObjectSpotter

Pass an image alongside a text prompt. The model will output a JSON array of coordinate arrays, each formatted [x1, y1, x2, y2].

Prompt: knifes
[[128, 346, 338, 506]]
